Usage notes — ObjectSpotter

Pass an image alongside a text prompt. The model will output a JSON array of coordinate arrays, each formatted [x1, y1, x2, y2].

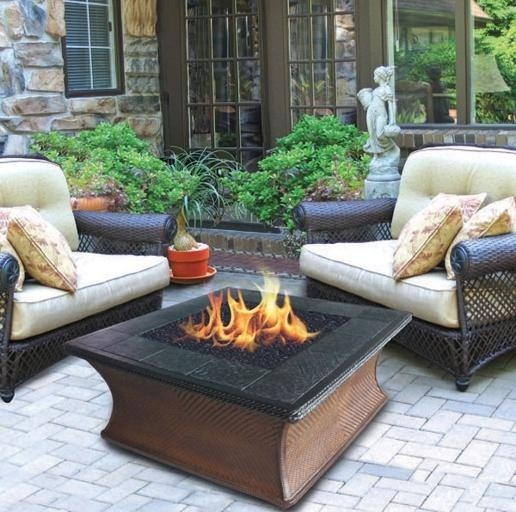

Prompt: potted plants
[[69, 161, 128, 213], [160, 147, 244, 285]]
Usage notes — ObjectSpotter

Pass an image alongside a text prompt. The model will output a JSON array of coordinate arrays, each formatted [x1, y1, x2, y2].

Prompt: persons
[[363, 66, 395, 165], [425, 67, 454, 122]]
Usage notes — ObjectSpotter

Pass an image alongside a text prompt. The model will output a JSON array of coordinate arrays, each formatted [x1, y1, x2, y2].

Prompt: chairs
[[0, 154, 178, 403], [288, 143, 516, 392]]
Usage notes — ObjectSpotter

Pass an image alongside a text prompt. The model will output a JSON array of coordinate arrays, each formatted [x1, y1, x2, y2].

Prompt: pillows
[[0, 205, 77, 295], [390, 193, 515, 282]]
